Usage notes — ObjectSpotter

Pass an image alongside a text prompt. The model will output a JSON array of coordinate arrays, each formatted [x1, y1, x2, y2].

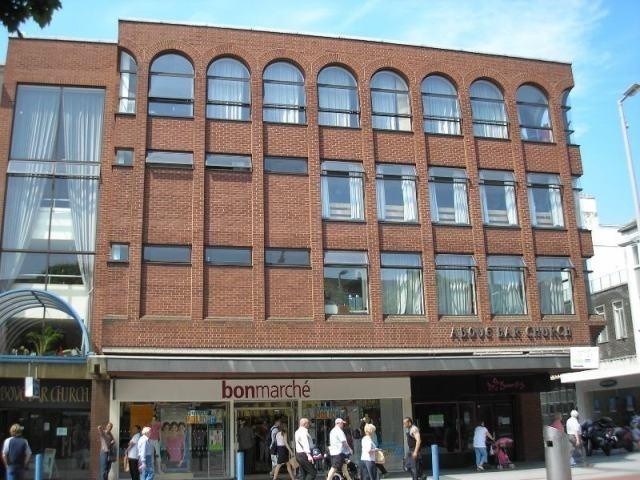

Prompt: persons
[[473, 420, 493, 472], [161, 421, 188, 468], [327, 417, 351, 480], [148, 417, 165, 474], [403, 416, 421, 480], [360, 423, 380, 480], [295, 418, 317, 480], [126, 424, 154, 480], [97, 423, 116, 480], [550, 410, 565, 434], [565, 409, 592, 467], [361, 413, 373, 429], [496, 433, 511, 467], [238, 417, 295, 480], [1, 423, 33, 480]]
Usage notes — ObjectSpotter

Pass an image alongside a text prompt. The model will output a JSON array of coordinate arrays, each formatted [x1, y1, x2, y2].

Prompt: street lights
[[615, 82, 640, 247], [338, 269, 348, 291]]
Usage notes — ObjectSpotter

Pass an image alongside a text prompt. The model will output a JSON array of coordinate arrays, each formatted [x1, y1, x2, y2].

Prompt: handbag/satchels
[[124, 452, 129, 472], [289, 453, 299, 469], [374, 450, 385, 464], [269, 434, 277, 454]]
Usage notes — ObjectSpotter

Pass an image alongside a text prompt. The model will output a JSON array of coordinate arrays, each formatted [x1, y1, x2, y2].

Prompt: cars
[[581, 416, 634, 456]]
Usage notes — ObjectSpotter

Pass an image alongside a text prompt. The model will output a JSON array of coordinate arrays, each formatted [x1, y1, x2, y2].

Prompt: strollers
[[492, 437, 515, 470]]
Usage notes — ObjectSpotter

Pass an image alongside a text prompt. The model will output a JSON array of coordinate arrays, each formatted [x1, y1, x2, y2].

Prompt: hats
[[335, 417, 346, 424]]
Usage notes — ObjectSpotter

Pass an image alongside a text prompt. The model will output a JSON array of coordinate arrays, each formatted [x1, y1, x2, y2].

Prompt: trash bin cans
[[543, 425, 573, 480]]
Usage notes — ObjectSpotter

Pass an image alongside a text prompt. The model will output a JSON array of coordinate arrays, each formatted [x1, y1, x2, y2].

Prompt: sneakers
[[479, 466, 484, 470]]
